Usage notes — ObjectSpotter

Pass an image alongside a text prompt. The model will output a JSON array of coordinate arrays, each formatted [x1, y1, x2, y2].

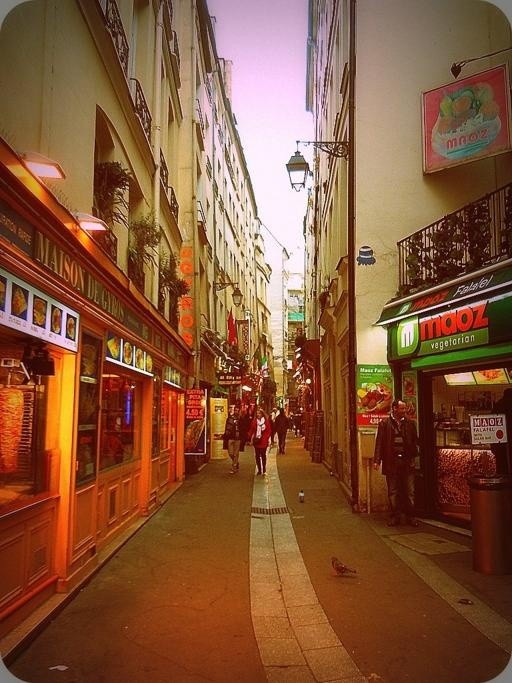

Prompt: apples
[[479, 100, 499, 121], [438, 117, 452, 134], [473, 82, 493, 105]]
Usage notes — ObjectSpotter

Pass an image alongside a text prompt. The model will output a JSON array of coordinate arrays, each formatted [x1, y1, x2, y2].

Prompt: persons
[[268, 406, 302, 454], [247, 409, 272, 476], [223, 405, 246, 474], [372, 400, 419, 527]]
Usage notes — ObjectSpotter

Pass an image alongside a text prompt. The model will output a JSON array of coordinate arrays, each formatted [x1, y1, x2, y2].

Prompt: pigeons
[[330, 556, 357, 578], [298, 490, 305, 503]]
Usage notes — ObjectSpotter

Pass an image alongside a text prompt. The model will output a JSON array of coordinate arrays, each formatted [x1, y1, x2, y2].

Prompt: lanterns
[[285, 151, 309, 192]]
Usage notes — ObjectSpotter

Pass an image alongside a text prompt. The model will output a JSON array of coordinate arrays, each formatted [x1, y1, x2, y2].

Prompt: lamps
[[286, 140, 352, 191], [212, 281, 243, 308], [73, 212, 110, 231], [450, 46, 512, 79], [22, 150, 67, 180]]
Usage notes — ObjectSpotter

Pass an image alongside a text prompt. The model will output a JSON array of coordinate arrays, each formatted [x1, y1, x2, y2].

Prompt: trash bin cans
[[467, 475, 512, 575]]
